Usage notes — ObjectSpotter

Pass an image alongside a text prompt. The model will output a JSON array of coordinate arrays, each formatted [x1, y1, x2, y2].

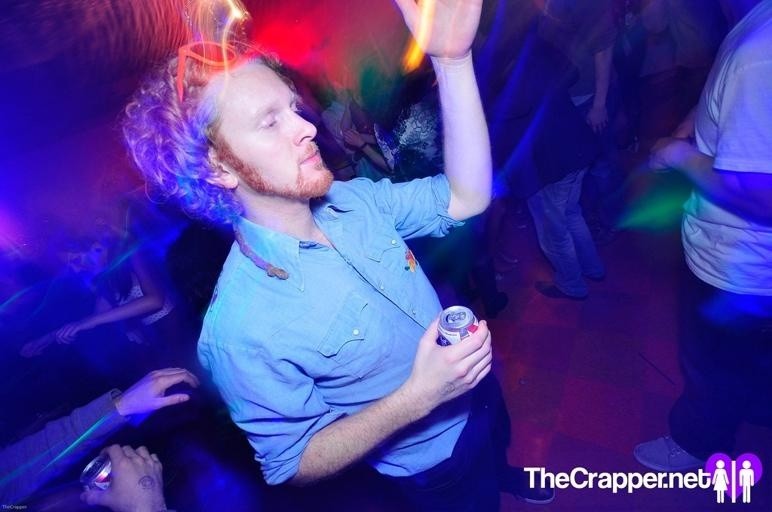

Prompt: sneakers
[[534, 279, 590, 301], [500, 463, 558, 506], [475, 291, 509, 319], [585, 275, 606, 285], [631, 434, 708, 474]]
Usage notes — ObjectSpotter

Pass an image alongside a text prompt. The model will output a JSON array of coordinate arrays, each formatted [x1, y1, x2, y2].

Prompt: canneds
[[79, 457, 113, 495], [437, 304, 478, 346]]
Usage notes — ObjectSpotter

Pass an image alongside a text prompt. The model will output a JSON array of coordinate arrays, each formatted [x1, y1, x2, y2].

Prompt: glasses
[[173, 37, 269, 104]]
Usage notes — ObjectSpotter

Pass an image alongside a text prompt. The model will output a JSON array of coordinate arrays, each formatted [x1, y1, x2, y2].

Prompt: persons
[[18, 226, 180, 358], [0, 365, 200, 511], [120, 0, 557, 511], [274, 2, 770, 475]]
